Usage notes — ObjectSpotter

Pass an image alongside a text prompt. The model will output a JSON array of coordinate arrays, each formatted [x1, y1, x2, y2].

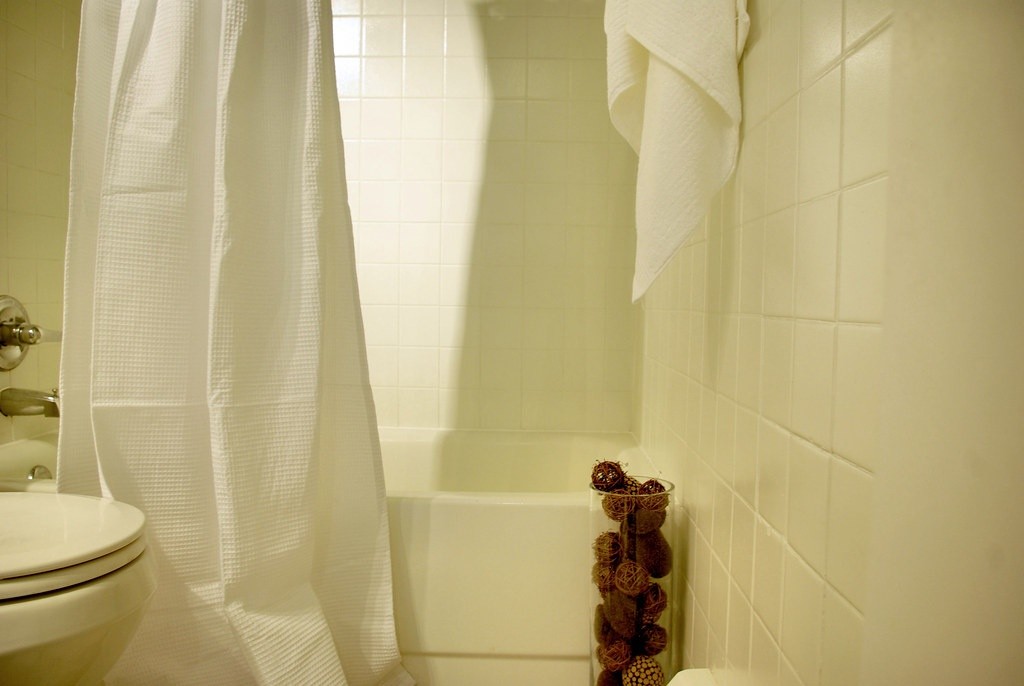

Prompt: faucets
[[1, 386, 60, 419]]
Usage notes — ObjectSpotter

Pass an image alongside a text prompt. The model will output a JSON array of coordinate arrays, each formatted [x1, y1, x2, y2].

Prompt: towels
[[602, 0, 752, 300]]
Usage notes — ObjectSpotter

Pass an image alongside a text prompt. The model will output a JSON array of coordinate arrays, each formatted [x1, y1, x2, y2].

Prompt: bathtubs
[[0, 425, 678, 683]]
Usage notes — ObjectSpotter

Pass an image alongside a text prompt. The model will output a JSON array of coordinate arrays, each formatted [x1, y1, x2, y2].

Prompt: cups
[[590, 476, 673, 686]]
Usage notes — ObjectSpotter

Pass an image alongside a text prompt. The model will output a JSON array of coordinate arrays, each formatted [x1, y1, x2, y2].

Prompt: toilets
[[0, 489, 156, 685]]
